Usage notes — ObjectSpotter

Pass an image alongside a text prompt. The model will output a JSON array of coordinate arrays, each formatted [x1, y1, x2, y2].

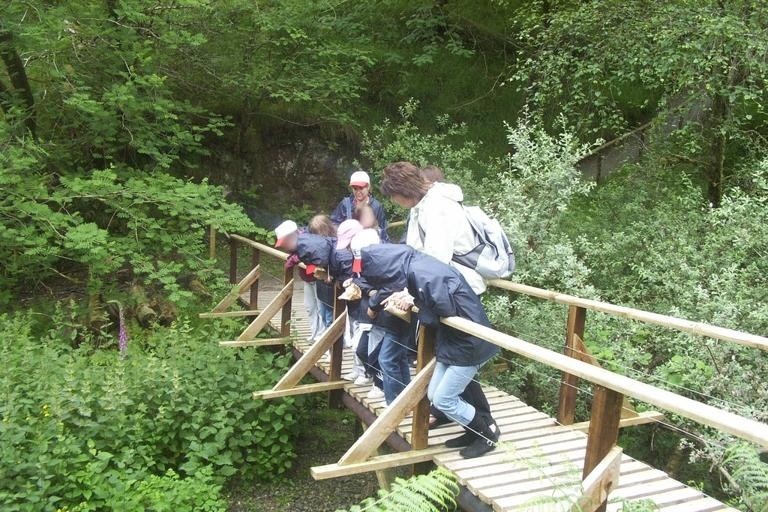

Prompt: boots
[[445, 408, 500, 459]]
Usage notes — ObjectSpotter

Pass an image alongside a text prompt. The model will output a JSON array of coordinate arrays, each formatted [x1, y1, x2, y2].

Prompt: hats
[[336, 218, 363, 250], [274, 220, 298, 249], [349, 171, 370, 188], [350, 228, 380, 272]]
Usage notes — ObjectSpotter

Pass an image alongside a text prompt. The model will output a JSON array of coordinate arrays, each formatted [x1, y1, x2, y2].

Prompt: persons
[[381, 161, 492, 415], [274, 216, 501, 457], [398, 165, 444, 244], [330, 171, 389, 244]]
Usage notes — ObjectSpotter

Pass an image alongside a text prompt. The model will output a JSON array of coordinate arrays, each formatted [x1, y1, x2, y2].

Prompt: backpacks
[[418, 201, 515, 279]]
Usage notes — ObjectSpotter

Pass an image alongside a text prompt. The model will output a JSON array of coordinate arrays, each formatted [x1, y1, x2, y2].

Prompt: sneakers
[[343, 371, 374, 387], [367, 385, 385, 398], [429, 416, 452, 428]]
